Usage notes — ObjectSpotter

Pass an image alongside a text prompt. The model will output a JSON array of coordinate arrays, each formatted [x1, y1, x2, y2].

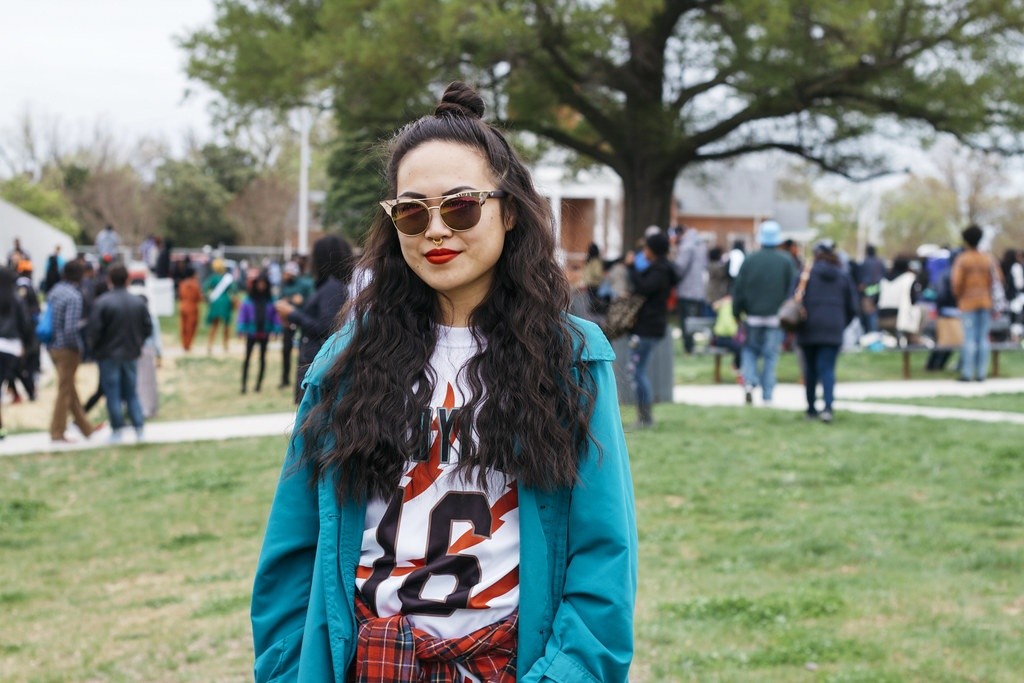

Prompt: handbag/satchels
[[778, 301, 806, 331]]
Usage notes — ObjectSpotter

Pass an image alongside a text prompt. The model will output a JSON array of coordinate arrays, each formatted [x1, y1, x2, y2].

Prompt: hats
[[754, 221, 786, 247]]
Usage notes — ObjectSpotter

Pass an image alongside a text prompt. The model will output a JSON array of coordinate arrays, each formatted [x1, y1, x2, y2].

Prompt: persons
[[670, 220, 805, 404], [877, 220, 1024, 382], [251, 81, 639, 683], [0, 222, 163, 442], [794, 239, 887, 423], [569, 226, 684, 430], [157, 235, 355, 405]]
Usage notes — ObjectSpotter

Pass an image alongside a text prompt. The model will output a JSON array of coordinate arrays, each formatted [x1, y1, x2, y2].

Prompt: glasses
[[388, 190, 509, 238]]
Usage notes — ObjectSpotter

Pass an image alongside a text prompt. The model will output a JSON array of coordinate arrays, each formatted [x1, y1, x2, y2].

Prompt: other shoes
[[84, 422, 104, 437], [745, 385, 753, 403], [820, 410, 833, 422]]
